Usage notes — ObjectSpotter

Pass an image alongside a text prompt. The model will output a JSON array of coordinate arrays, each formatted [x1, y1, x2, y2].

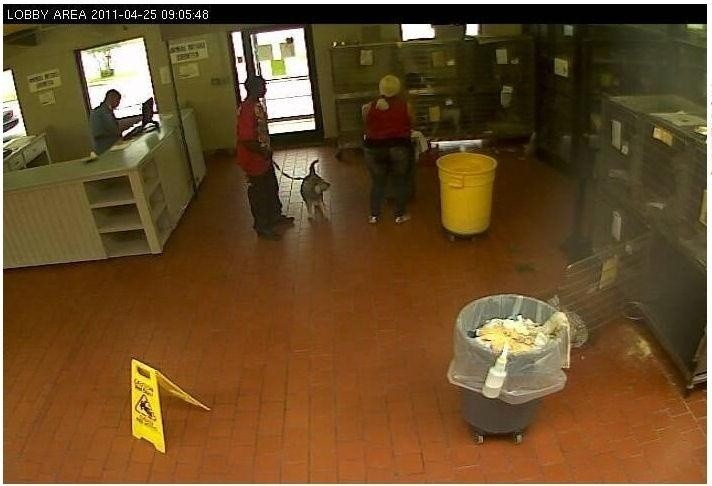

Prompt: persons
[[360, 74, 418, 225], [88, 88, 123, 156], [234, 74, 295, 239]]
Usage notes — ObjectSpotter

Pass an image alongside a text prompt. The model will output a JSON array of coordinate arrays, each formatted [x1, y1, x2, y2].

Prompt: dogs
[[422, 102, 461, 137], [300, 159, 331, 220]]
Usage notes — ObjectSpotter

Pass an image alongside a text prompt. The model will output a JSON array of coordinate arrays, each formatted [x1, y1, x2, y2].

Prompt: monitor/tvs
[[141, 97, 159, 133]]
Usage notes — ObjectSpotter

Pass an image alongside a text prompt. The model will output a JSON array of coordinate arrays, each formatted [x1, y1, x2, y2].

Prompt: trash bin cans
[[447, 293, 570, 444], [436, 153, 498, 243]]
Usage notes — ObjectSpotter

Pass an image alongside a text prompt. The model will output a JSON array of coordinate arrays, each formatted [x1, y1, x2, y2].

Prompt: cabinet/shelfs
[[560, 95, 707, 398], [329, 37, 537, 163], [525, 24, 673, 173]]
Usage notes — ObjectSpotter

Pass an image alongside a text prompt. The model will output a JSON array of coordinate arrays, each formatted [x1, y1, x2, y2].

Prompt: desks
[[3, 133, 51, 172]]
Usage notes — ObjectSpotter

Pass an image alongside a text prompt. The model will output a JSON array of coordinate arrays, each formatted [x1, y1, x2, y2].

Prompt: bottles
[[481, 355, 508, 399]]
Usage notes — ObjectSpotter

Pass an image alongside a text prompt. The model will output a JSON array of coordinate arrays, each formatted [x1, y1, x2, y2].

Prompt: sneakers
[[396, 214, 411, 223], [370, 216, 377, 224], [259, 229, 280, 240], [272, 215, 294, 224]]
